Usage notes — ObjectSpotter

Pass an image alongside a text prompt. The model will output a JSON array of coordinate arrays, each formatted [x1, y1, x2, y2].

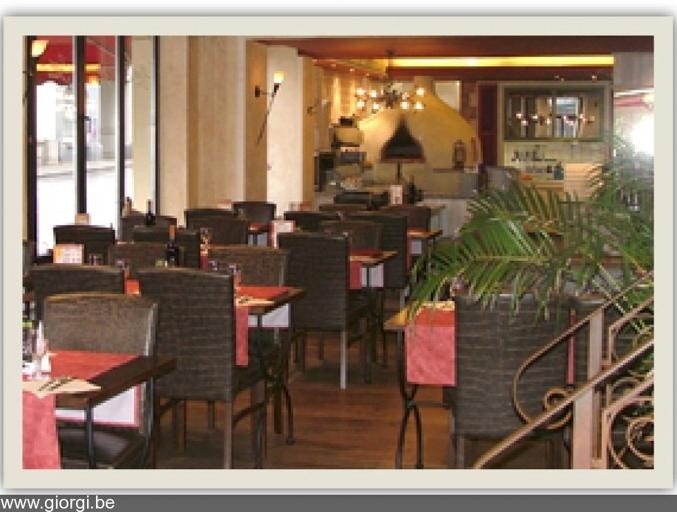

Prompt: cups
[[34, 338, 52, 379]]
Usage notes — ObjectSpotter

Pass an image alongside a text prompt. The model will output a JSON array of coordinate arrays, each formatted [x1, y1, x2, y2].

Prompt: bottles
[[144, 201, 156, 227], [23, 299, 36, 363], [166, 223, 180, 270]]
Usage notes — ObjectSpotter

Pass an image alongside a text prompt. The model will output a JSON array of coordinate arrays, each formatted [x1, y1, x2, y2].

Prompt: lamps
[[354, 47, 428, 111], [375, 112, 431, 165], [255, 70, 283, 97]]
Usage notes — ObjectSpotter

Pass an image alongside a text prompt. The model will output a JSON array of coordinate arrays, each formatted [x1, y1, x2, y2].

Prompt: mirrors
[[506, 88, 604, 140]]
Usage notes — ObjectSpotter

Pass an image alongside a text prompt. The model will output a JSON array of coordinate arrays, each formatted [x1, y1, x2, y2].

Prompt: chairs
[[20, 185, 652, 470]]
